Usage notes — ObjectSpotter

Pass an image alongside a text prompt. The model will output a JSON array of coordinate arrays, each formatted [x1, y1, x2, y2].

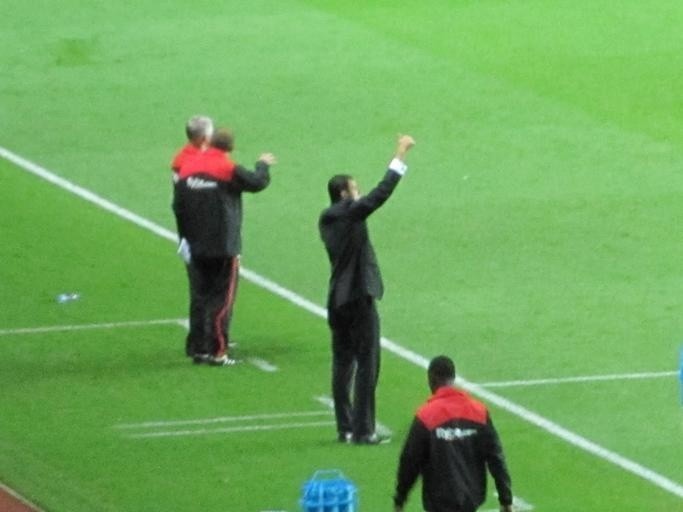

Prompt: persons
[[172, 127, 276, 366], [392, 354, 513, 512], [317, 134, 416, 446], [169, 116, 238, 353]]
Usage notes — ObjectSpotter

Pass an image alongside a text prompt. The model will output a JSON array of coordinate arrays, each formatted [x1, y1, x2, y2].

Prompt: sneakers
[[194, 353, 237, 366], [339, 431, 390, 443]]
